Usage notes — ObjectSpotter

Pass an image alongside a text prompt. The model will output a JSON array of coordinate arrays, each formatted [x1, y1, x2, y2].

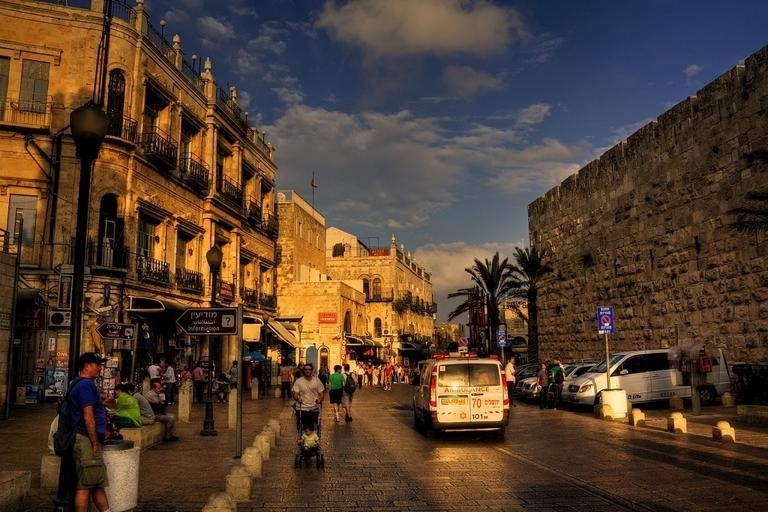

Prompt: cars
[[508, 359, 602, 404]]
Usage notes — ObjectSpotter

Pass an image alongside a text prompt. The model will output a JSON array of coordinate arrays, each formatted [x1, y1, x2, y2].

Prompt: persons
[[105, 350, 270, 441], [279, 359, 418, 462], [51, 353, 110, 511], [505, 357, 565, 411]]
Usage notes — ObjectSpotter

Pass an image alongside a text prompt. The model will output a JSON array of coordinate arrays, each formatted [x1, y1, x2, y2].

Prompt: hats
[[78, 352, 107, 366]]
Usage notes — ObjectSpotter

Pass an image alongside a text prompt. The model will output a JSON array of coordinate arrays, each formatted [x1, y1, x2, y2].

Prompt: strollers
[[291, 399, 327, 470]]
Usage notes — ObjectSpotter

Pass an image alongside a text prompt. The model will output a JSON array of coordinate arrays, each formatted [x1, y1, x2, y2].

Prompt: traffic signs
[[96, 322, 136, 341], [174, 307, 238, 336]]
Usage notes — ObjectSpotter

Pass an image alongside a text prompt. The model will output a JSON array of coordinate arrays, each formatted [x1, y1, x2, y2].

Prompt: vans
[[565, 345, 734, 410], [410, 350, 513, 442]]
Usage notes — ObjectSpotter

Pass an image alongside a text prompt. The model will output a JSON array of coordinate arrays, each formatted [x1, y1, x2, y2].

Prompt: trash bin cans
[[89, 439, 141, 512]]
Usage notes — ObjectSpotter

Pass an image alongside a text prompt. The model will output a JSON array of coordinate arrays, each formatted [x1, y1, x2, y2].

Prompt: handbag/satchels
[[52, 428, 76, 455]]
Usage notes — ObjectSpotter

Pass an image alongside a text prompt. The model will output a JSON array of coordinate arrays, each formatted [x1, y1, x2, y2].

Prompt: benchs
[[0, 422, 166, 512]]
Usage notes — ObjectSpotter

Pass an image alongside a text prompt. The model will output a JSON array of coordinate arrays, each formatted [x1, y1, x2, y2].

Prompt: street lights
[[198, 241, 224, 437], [61, 95, 113, 388], [298, 323, 303, 363]]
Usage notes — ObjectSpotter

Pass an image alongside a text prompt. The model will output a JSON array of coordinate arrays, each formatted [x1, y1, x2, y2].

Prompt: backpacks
[[342, 372, 355, 393]]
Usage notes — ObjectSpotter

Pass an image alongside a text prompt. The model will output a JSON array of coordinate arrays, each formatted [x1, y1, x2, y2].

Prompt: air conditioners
[[382, 329, 390, 336], [49, 312, 71, 327]]
